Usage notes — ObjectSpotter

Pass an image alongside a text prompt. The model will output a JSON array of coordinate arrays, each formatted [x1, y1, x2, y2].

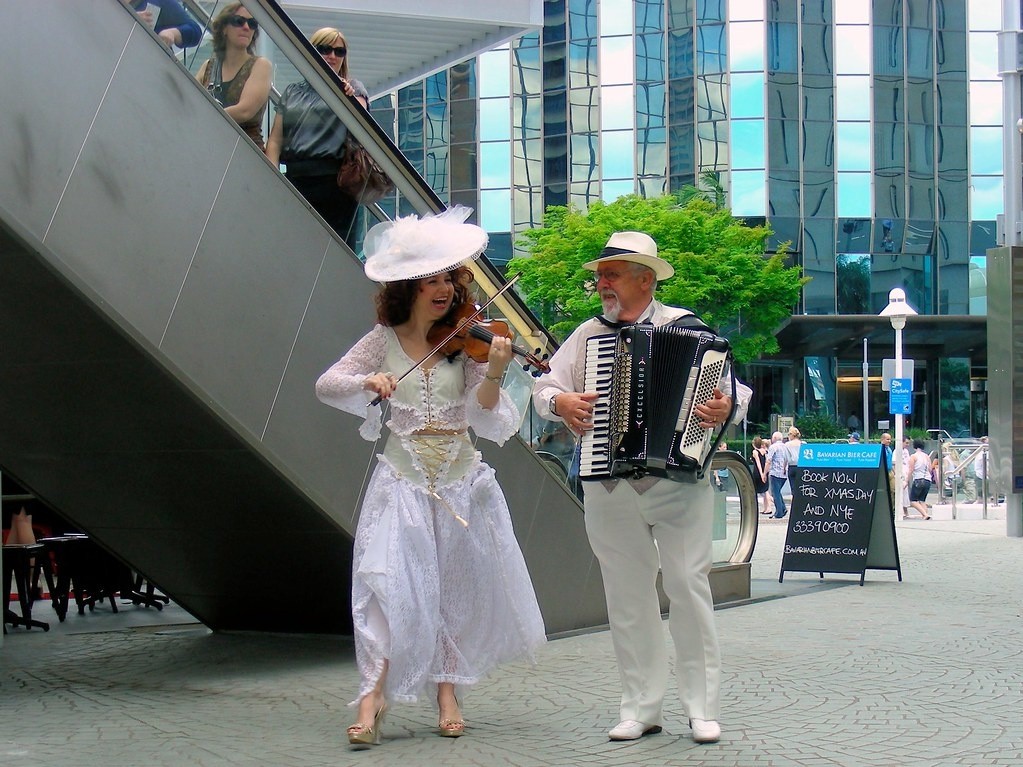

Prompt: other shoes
[[757, 500, 945, 525]]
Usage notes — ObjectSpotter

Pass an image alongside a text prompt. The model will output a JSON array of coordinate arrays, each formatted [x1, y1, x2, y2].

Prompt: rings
[[386, 372, 393, 379], [496, 347, 500, 350], [569, 424, 573, 427], [714, 416, 716, 421]]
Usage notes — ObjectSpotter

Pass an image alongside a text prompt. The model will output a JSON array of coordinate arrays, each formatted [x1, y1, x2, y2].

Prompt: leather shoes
[[687, 716, 722, 743], [606, 719, 663, 740]]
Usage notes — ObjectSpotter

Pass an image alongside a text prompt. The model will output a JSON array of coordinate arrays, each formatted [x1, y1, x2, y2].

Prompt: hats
[[362, 205, 489, 283], [582, 231, 675, 281]]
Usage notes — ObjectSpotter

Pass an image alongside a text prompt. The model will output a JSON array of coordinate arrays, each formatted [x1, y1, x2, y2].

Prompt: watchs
[[486, 373, 503, 383], [549, 393, 562, 415]]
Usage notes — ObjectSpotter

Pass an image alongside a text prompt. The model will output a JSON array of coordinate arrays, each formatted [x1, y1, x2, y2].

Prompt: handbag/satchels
[[337, 145, 393, 207]]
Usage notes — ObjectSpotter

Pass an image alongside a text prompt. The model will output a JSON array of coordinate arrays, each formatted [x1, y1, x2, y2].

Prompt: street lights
[[877, 286, 919, 531]]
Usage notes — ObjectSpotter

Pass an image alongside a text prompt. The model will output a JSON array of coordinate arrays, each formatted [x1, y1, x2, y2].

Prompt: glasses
[[593, 269, 630, 282], [227, 14, 258, 30], [315, 44, 347, 57]]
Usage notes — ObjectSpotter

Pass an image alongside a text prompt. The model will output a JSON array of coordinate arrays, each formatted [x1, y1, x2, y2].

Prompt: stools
[[2, 535, 169, 635]]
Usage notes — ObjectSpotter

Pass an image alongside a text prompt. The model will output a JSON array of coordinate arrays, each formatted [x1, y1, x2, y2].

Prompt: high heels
[[436, 692, 465, 737], [347, 695, 388, 745]]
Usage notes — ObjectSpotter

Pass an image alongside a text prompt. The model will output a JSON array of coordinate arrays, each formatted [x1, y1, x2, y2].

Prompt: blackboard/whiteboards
[[782, 443, 900, 573]]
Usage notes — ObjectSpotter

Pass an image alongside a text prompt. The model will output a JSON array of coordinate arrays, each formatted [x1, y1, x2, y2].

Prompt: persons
[[124, 0, 202, 53], [881, 433, 895, 511], [315, 204, 547, 745], [941, 436, 989, 504], [5, 479, 44, 595], [714, 441, 728, 492], [195, 2, 273, 153], [752, 426, 807, 519], [880, 219, 895, 252], [266, 28, 370, 250], [892, 435, 910, 516], [533, 229, 753, 742], [847, 411, 858, 431], [903, 438, 932, 520], [847, 432, 860, 444]]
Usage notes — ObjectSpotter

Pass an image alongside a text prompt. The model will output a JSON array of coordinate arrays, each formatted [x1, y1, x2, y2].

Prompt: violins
[[425, 301, 554, 380]]
